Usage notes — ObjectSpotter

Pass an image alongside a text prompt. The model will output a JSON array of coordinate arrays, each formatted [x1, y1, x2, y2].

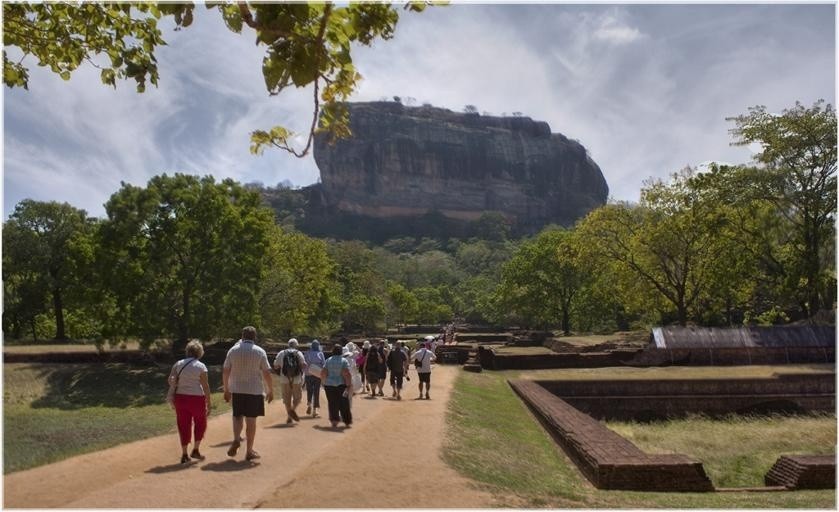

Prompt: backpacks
[[282, 350, 302, 377]]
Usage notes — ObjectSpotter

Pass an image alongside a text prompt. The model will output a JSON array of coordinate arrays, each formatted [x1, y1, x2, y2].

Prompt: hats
[[345, 342, 357, 350], [342, 347, 354, 358], [288, 338, 298, 348], [362, 341, 372, 349]]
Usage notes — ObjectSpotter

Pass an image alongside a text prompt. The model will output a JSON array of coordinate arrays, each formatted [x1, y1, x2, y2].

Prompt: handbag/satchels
[[308, 364, 321, 378], [167, 385, 177, 411], [414, 358, 423, 369]]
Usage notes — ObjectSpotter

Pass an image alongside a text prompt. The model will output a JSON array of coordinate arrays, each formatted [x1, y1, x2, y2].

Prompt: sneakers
[[228, 440, 240, 456], [286, 407, 300, 424], [306, 406, 322, 419], [181, 454, 191, 464], [362, 384, 402, 401], [419, 392, 430, 399], [191, 448, 205, 460], [246, 451, 260, 460]]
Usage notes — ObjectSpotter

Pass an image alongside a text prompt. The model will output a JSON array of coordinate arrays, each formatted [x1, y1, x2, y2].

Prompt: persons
[[166, 336, 212, 465], [221, 325, 273, 461], [274, 321, 457, 429]]
